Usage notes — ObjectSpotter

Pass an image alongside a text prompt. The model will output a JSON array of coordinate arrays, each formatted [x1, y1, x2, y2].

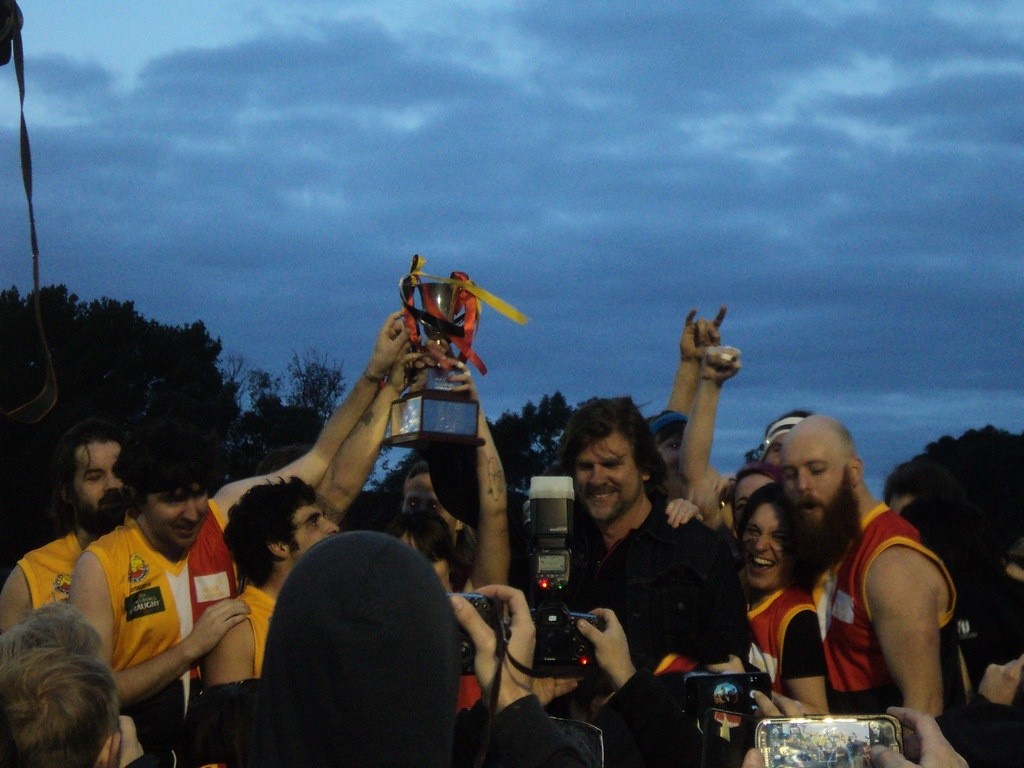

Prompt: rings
[[458, 361, 464, 368]]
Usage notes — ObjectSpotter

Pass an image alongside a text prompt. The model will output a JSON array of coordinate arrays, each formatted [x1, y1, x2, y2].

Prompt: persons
[[0, 599, 162, 768], [0, 309, 425, 758], [558, 305, 1024, 768], [401, 359, 510, 713], [172, 531, 604, 768]]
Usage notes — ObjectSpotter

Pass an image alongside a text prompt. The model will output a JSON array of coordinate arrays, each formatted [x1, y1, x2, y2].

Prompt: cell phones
[[683, 673, 773, 717], [754, 713, 904, 768], [702, 707, 758, 768]]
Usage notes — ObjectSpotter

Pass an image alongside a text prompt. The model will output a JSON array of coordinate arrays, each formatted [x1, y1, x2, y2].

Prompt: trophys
[[382, 254, 527, 453]]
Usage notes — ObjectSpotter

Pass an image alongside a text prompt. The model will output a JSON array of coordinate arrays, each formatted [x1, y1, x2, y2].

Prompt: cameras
[[443, 591, 508, 675], [524, 476, 606, 678]]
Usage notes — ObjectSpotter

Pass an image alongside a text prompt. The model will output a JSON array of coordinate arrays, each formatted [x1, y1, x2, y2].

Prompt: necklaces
[[590, 527, 635, 581]]
